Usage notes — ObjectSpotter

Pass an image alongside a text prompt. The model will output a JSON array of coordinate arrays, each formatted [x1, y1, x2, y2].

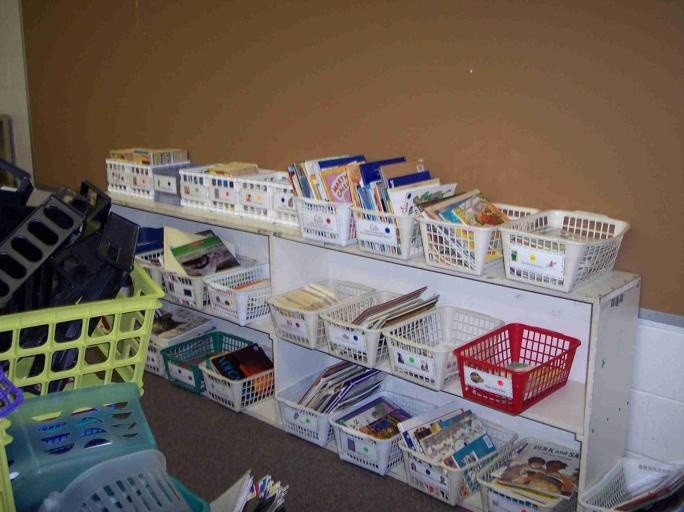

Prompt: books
[[135, 308, 210, 370], [286, 154, 522, 271], [108, 148, 188, 194], [612, 462, 683, 510], [509, 226, 621, 285], [396, 400, 504, 501], [210, 468, 289, 511], [271, 283, 347, 343], [405, 343, 469, 384], [330, 286, 441, 359], [206, 342, 275, 408], [287, 361, 387, 441], [158, 224, 271, 311], [337, 395, 413, 468], [488, 441, 580, 511], [203, 162, 259, 209], [492, 362, 564, 399], [179, 350, 232, 388]]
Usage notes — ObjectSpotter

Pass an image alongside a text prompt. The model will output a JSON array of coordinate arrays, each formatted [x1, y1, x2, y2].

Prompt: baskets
[[580, 457, 683, 511], [382, 307, 506, 392], [105, 160, 191, 200], [349, 205, 425, 262], [1, 255, 210, 512], [275, 370, 390, 447], [0, 260, 213, 512], [476, 437, 580, 512], [398, 416, 518, 506], [236, 170, 299, 228], [295, 196, 358, 247], [319, 288, 441, 369], [133, 247, 270, 326], [180, 165, 275, 215], [265, 279, 377, 349], [279, 368, 577, 511], [415, 202, 541, 276], [330, 391, 440, 475], [498, 209, 630, 293], [129, 315, 275, 413], [265, 279, 583, 415], [105, 148, 631, 293], [453, 323, 581, 416]]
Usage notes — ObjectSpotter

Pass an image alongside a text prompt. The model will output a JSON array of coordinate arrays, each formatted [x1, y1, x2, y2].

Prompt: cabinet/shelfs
[[105, 191, 640, 512]]
